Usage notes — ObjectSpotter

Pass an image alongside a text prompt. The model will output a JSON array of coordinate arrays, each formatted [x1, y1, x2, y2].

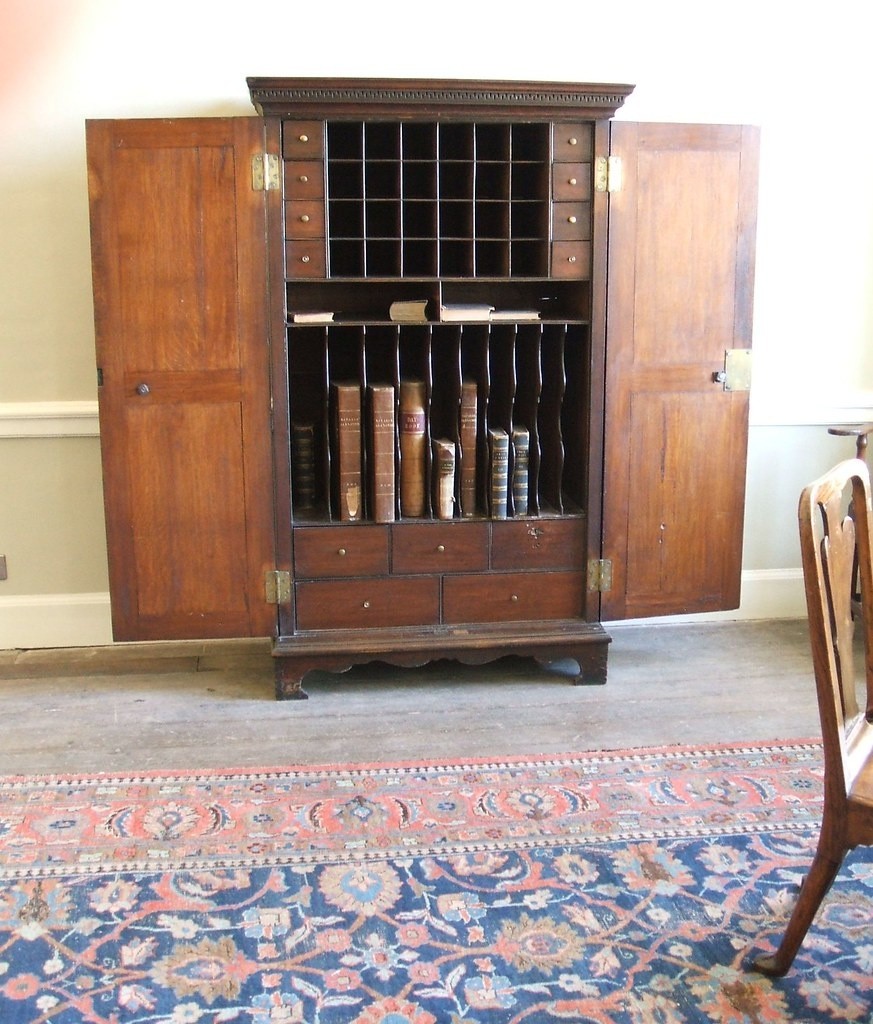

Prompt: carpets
[[0, 739, 872, 1024]]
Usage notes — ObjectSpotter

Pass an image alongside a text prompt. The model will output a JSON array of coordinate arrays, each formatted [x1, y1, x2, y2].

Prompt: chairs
[[769, 419, 873, 979]]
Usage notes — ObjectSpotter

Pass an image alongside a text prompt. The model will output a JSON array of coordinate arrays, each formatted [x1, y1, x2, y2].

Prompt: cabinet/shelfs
[[84, 76, 762, 699]]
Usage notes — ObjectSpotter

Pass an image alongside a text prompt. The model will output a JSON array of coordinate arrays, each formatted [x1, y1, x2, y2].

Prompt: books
[[488, 426, 510, 517], [388, 299, 429, 322], [491, 310, 542, 320], [288, 309, 335, 323], [399, 379, 428, 517], [330, 377, 362, 523], [432, 437, 455, 522], [442, 302, 496, 321], [290, 412, 318, 510], [514, 424, 529, 515], [366, 383, 396, 525], [462, 380, 478, 517]]
